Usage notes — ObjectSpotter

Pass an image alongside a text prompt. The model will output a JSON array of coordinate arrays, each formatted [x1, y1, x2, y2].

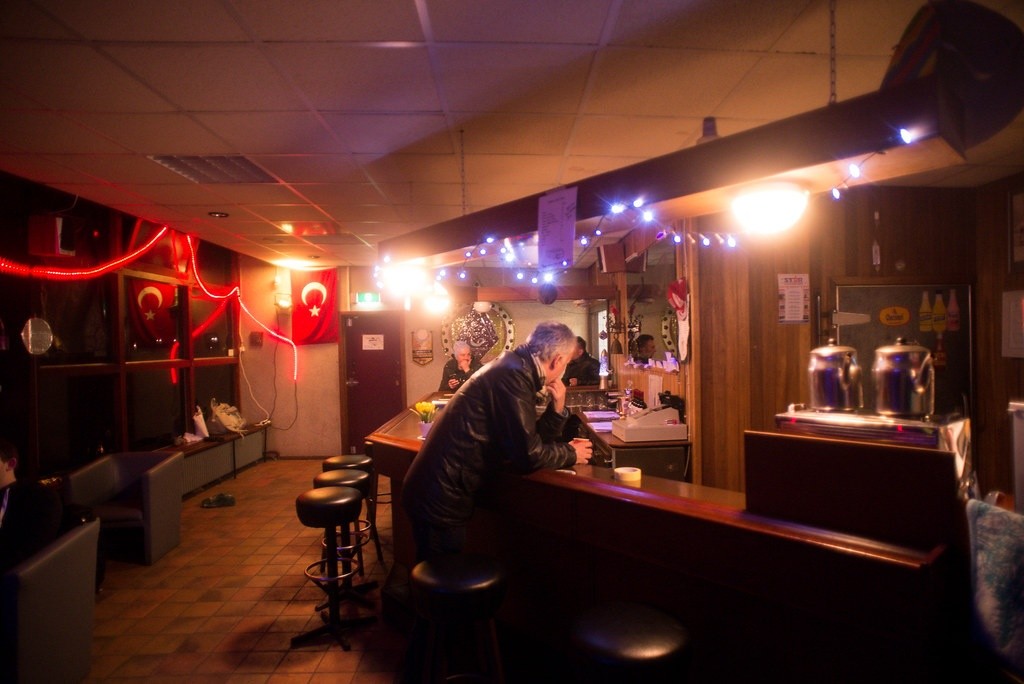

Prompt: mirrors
[[623, 230, 681, 376]]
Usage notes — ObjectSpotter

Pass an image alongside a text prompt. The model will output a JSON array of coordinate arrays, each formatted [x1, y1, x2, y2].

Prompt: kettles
[[872, 338, 940, 419], [805, 338, 864, 410]]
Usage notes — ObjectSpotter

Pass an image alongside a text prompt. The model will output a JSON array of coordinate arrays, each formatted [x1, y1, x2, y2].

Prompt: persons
[[438, 341, 484, 391], [401, 320, 593, 684], [0, 430, 34, 569], [634, 334, 656, 365], [561, 336, 601, 387]]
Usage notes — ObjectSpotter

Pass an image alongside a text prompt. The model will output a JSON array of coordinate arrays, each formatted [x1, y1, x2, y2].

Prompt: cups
[[574, 438, 589, 443]]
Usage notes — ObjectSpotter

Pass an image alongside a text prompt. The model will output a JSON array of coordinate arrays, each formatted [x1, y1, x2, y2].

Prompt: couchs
[[68, 449, 185, 566], [0, 515, 101, 683]]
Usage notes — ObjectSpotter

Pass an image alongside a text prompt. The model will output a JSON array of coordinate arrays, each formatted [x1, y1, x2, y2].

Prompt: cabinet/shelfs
[[575, 411, 692, 481]]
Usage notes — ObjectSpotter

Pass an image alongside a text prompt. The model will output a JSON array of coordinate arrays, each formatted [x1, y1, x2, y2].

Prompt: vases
[[416, 420, 434, 440]]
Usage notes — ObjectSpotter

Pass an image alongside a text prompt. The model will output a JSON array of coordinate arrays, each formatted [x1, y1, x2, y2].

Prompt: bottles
[[947, 290, 961, 330], [935, 336, 946, 372], [627, 389, 646, 414], [932, 290, 947, 332], [919, 292, 932, 332]]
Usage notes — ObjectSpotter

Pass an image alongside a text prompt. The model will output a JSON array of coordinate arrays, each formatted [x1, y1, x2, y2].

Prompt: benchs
[[151, 418, 272, 496]]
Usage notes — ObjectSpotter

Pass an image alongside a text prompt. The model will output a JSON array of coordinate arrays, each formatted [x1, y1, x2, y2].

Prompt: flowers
[[409, 401, 439, 422]]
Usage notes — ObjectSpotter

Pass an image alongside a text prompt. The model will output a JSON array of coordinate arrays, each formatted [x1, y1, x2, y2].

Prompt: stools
[[408, 552, 509, 684], [317, 454, 385, 577], [289, 485, 376, 651], [310, 466, 380, 611], [568, 604, 686, 684]]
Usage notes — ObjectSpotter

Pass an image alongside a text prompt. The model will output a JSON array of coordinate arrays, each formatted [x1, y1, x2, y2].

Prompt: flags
[[125, 277, 179, 344], [290, 267, 342, 346]]
